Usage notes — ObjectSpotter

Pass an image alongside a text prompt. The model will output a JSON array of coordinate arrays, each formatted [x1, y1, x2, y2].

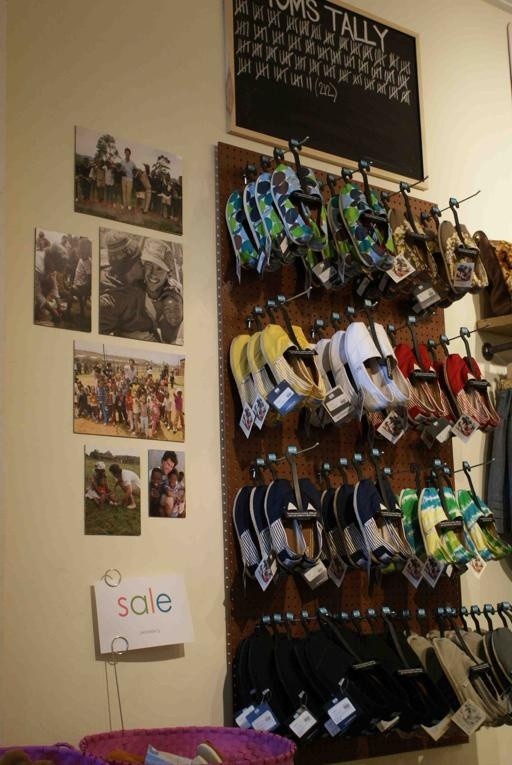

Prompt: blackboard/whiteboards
[[223, 0, 428, 191]]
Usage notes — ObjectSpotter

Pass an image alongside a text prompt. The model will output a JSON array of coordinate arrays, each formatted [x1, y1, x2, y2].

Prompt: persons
[[36, 146, 185, 341], [74, 346, 186, 516]]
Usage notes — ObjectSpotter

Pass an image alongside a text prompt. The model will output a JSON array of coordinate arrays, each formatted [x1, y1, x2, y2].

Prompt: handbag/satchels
[[473, 230, 512, 315]]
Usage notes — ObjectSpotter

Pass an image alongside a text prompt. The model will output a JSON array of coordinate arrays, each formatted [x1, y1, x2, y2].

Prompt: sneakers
[[143, 739, 224, 765], [234, 479, 511, 579], [224, 162, 490, 301], [233, 627, 512, 737], [230, 322, 501, 432]]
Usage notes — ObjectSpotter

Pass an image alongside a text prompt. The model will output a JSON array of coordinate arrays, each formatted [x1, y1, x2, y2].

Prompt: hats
[[104, 232, 141, 274], [49, 242, 69, 272], [94, 462, 106, 472], [140, 238, 174, 271]]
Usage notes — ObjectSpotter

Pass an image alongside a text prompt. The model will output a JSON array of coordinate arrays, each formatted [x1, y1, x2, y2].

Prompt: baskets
[[79, 726, 297, 765], [0, 742, 109, 765]]
